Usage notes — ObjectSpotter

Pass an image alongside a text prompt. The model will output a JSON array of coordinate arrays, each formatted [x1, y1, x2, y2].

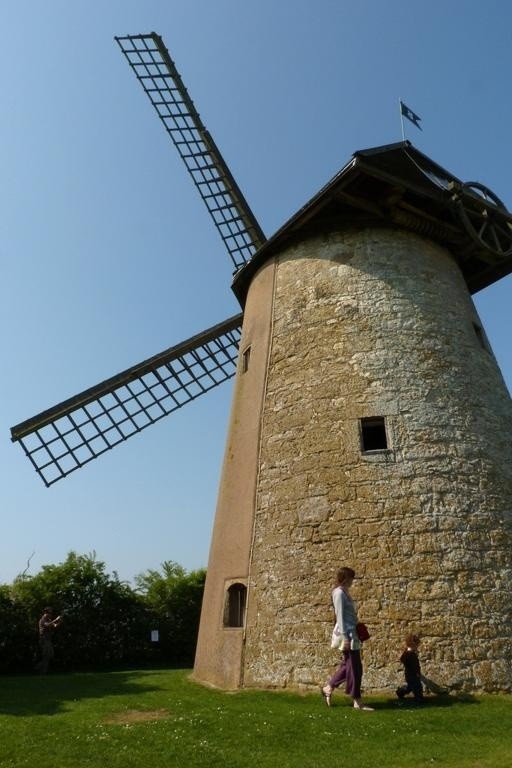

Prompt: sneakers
[[322, 686, 333, 706], [354, 703, 375, 711]]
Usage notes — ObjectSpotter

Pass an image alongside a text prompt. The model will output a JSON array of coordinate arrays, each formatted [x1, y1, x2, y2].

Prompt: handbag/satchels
[[357, 622, 370, 641]]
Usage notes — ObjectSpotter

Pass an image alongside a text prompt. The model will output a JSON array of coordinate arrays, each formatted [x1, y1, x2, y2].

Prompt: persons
[[35, 606, 64, 675], [396, 634, 425, 704], [322, 567, 376, 712]]
